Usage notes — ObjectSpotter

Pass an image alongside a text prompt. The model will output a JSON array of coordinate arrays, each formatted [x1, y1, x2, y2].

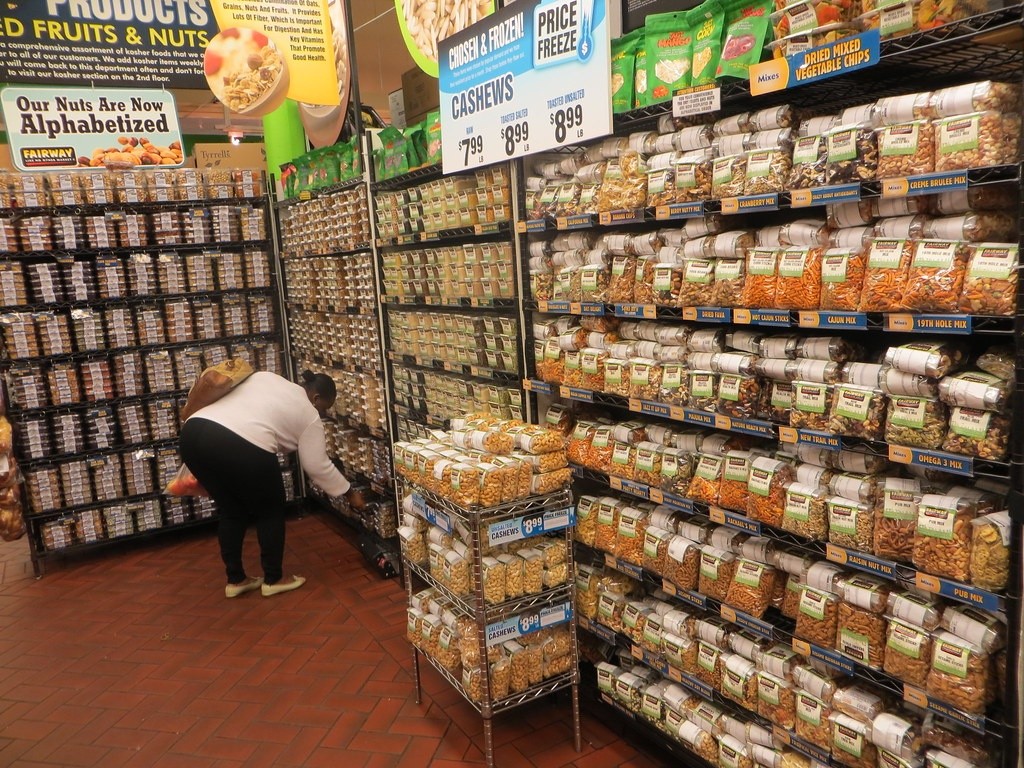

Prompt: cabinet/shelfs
[[360, 128, 532, 437], [269, 153, 407, 593], [521, 0, 1024, 768], [0, 169, 310, 581], [391, 411, 581, 768]]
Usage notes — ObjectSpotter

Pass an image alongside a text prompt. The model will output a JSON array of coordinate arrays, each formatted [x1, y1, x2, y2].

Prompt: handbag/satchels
[[179, 356, 254, 422], [162, 463, 209, 496]]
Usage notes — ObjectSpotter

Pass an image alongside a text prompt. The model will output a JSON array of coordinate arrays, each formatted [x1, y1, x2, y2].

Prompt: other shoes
[[261, 575, 306, 597], [225, 576, 264, 597]]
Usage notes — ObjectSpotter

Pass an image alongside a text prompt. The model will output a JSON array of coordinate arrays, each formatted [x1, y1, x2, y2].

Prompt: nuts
[[530, 80, 1020, 768], [393, 413, 577, 707]]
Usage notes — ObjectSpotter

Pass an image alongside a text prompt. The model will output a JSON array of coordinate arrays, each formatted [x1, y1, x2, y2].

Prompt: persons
[[178, 369, 364, 599]]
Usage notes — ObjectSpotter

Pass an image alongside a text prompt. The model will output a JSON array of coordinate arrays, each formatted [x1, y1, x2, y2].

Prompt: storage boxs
[[388, 64, 440, 130], [191, 143, 271, 180]]
